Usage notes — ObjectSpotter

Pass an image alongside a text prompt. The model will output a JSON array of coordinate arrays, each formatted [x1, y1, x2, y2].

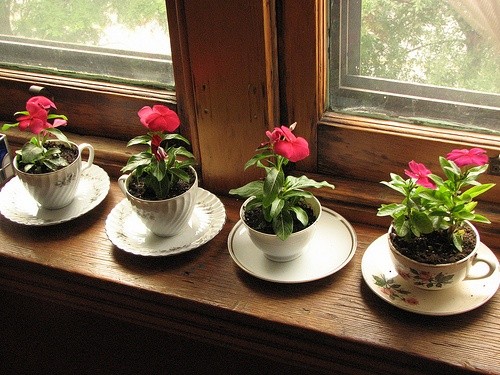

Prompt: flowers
[[120, 104, 199, 200], [2, 96, 70, 172], [228, 122, 336, 241], [376, 148, 496, 253]]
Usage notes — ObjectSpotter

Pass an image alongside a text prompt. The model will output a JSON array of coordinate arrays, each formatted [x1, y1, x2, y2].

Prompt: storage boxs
[[0, 134, 15, 187]]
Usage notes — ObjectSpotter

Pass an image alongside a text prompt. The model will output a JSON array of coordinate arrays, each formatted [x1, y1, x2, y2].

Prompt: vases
[[239, 194, 322, 263], [13, 139, 95, 209], [386, 220, 496, 291], [118, 160, 198, 236]]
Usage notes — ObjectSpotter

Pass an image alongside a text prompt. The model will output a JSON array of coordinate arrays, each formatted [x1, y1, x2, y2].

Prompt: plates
[[103, 187, 227, 257], [0, 161, 110, 225], [226, 205, 358, 284], [360, 232, 500, 316]]
